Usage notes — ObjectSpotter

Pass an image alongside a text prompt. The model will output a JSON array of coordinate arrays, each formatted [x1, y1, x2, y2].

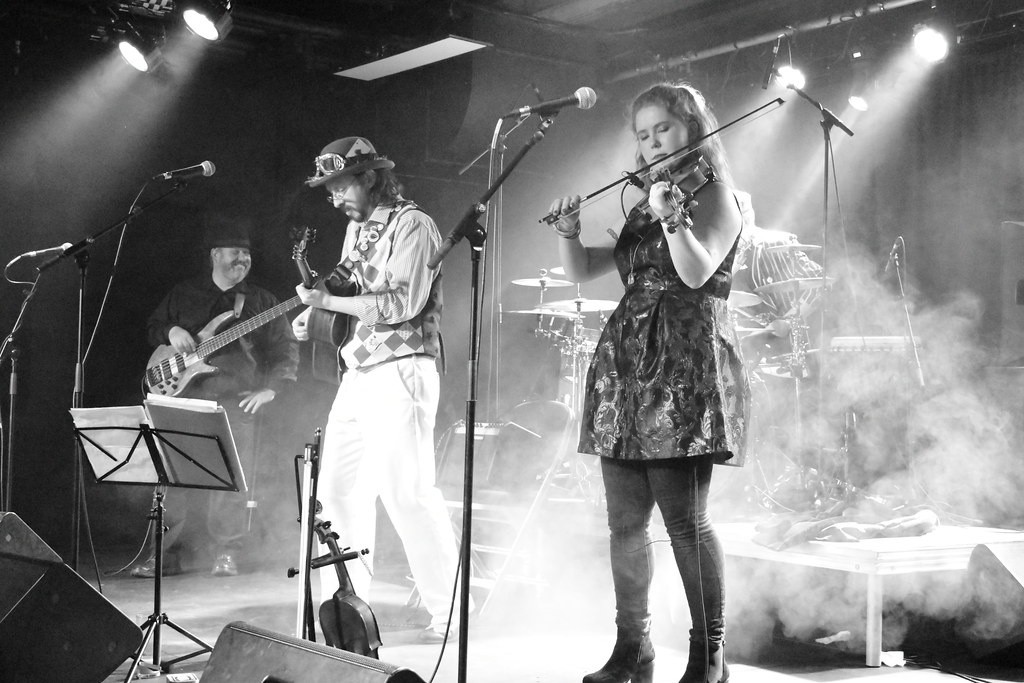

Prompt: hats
[[307, 137, 396, 188], [205, 222, 260, 253]]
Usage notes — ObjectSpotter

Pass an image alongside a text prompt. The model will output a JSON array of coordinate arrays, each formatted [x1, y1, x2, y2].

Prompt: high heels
[[584, 629, 656, 682], [677, 637, 731, 683]]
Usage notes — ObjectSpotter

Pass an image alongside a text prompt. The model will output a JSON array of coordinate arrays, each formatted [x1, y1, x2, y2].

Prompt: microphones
[[884, 237, 900, 273], [503, 86, 597, 120], [21, 243, 73, 259], [762, 38, 780, 90], [531, 84, 544, 102], [154, 161, 216, 181]]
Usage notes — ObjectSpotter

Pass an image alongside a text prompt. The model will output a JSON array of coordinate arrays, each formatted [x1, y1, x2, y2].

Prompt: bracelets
[[553, 219, 581, 240]]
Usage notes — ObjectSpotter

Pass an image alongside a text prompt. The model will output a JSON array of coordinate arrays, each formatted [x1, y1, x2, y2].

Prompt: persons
[[549, 84, 751, 683], [291, 137, 475, 645], [130, 221, 301, 579], [730, 188, 828, 448]]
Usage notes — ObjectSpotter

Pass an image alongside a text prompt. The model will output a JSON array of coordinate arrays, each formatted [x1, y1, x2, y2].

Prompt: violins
[[297, 496, 383, 660], [635, 155, 717, 234]]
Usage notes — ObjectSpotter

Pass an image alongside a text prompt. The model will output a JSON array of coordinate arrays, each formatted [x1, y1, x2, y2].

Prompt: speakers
[[0, 511, 144, 683], [198, 621, 428, 683], [436, 421, 543, 491], [955, 541, 1024, 669]]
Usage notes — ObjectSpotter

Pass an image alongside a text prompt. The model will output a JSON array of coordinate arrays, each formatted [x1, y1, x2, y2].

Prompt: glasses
[[327, 175, 364, 205], [314, 153, 346, 178]]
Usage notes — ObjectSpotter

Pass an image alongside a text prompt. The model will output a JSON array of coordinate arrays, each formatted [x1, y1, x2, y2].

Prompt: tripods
[[762, 286, 891, 521], [70, 422, 240, 683]]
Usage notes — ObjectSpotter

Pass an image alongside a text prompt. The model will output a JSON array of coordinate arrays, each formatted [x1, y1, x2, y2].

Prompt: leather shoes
[[419, 622, 457, 645], [130, 552, 182, 576], [210, 549, 239, 577]]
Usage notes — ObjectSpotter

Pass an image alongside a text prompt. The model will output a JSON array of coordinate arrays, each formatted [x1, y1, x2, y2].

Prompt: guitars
[[145, 295, 302, 398], [292, 225, 357, 386]]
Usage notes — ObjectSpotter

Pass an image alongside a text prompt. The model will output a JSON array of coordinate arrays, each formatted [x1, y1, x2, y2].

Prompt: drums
[[560, 357, 591, 387], [561, 329, 602, 356], [825, 335, 921, 375], [548, 315, 583, 341]]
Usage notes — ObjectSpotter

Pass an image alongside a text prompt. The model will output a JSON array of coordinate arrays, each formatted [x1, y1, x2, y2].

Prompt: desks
[[712, 525, 1023, 667]]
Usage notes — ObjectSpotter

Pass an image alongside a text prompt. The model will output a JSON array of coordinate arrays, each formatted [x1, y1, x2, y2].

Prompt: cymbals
[[766, 239, 822, 253], [534, 298, 619, 313], [496, 309, 586, 320], [510, 277, 575, 287], [726, 290, 763, 307], [752, 277, 834, 294]]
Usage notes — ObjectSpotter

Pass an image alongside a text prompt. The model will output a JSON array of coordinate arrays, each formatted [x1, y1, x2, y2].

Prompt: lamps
[[182, 0, 234, 42], [912, 0, 958, 64], [848, 46, 879, 112], [119, 0, 188, 72]]
